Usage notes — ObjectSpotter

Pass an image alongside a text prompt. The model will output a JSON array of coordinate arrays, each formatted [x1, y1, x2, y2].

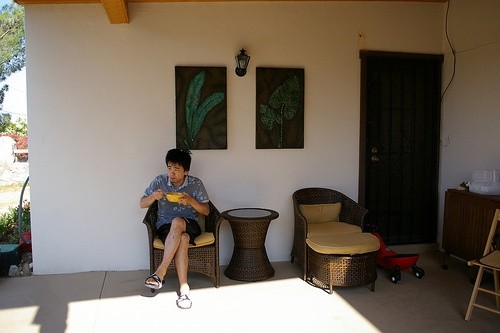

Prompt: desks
[[222, 207, 279, 282]]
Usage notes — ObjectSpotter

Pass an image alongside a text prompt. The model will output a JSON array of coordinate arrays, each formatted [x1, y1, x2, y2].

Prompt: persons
[[138, 148, 210, 309]]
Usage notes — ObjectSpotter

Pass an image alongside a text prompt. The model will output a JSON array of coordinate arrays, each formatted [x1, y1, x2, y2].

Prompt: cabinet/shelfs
[[439, 188, 500, 284]]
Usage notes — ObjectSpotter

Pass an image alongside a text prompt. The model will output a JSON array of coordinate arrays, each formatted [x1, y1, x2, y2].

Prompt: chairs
[[143, 199, 224, 288], [464, 209, 500, 322], [291, 187, 369, 280]]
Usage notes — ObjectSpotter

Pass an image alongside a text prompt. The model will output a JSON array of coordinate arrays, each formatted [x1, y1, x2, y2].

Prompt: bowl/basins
[[165, 192, 186, 202]]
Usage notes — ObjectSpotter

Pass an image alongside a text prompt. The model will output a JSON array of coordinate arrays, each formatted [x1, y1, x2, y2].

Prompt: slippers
[[176, 289, 192, 309], [144, 274, 166, 289]]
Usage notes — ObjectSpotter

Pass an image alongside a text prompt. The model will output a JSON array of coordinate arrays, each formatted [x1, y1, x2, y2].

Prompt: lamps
[[234, 48, 251, 77]]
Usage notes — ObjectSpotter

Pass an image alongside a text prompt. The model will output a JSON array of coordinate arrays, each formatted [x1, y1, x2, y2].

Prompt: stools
[[305, 233, 380, 293]]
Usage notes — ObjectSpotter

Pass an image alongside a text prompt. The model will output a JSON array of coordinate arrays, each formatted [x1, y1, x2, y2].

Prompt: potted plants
[[13, 206, 32, 252]]
